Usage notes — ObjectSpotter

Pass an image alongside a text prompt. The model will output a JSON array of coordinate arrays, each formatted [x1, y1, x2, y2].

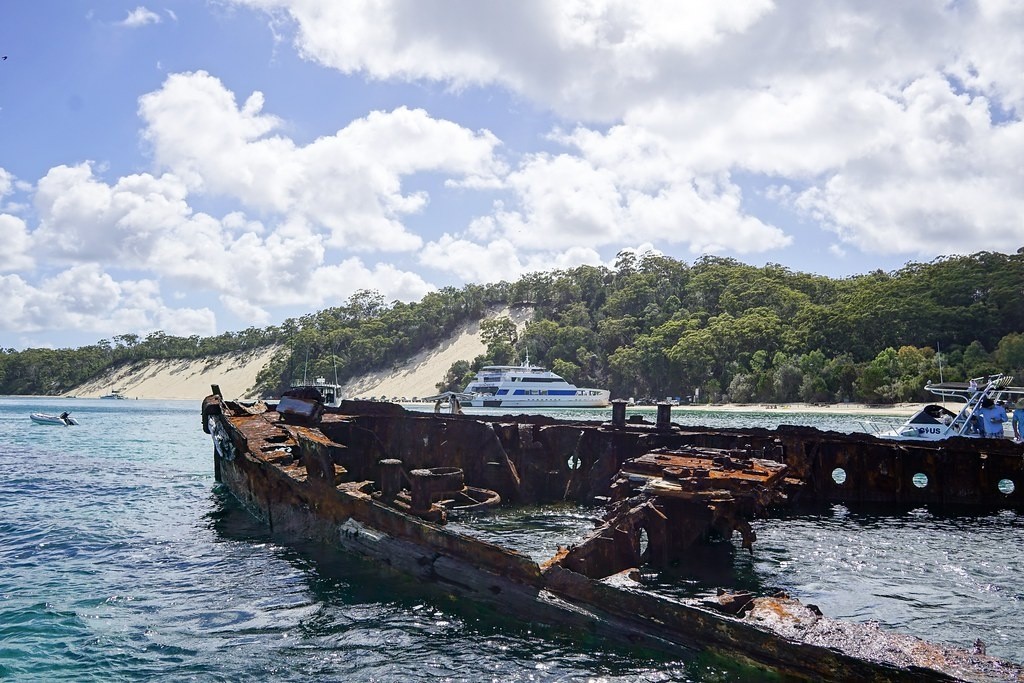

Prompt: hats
[[982, 399, 994, 408], [1015, 398, 1024, 409]]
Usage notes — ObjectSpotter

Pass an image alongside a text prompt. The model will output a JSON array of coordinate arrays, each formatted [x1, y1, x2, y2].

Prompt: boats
[[440, 347, 610, 407], [29, 411, 77, 426], [100, 388, 126, 401], [855, 376, 1024, 447]]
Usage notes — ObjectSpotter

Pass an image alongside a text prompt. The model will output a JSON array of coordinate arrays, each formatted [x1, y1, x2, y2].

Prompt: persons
[[958, 399, 1008, 439], [1000, 399, 1012, 412], [1013, 398, 1024, 441]]
